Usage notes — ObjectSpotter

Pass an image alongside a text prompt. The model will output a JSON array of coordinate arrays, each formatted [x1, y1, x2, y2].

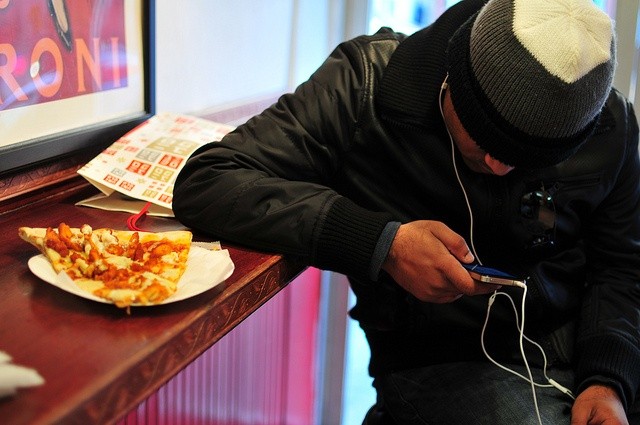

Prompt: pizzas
[[17, 226, 193, 309]]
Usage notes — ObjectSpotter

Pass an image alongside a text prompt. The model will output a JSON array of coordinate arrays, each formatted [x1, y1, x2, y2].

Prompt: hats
[[445, 0, 619, 169]]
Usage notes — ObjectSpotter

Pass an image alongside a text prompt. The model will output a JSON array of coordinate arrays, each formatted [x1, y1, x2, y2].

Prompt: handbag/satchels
[[76, 111, 236, 231]]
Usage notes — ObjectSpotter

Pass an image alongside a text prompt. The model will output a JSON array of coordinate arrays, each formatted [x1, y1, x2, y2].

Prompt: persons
[[171, 0, 639, 425]]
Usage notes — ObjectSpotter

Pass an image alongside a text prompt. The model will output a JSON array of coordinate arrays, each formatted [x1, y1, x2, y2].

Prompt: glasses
[[518, 169, 557, 248]]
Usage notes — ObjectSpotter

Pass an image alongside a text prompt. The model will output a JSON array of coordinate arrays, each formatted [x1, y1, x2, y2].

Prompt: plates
[[28, 246, 235, 306]]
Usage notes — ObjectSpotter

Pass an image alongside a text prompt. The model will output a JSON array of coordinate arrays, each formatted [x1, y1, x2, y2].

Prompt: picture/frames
[[0, 0, 156, 179]]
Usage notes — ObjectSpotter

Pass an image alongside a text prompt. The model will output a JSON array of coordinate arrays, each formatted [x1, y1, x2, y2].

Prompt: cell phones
[[460, 260, 526, 286]]
